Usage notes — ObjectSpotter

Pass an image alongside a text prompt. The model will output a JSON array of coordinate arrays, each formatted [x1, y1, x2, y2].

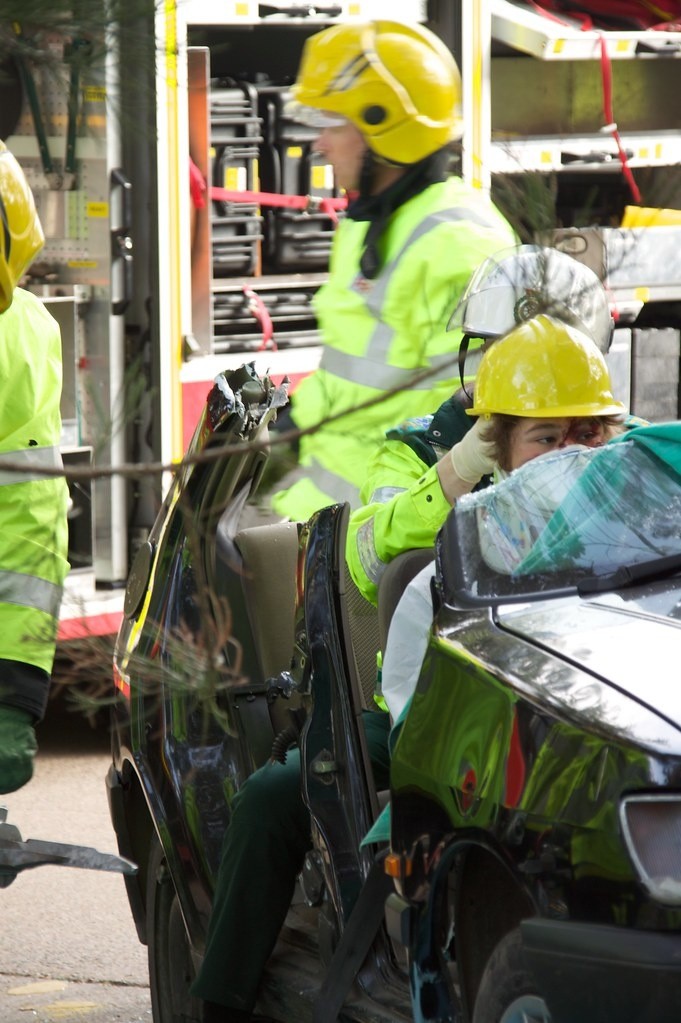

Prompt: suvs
[[100, 356, 680, 1023]]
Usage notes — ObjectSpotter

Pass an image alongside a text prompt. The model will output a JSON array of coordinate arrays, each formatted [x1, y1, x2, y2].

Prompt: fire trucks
[[0, 0, 681, 700]]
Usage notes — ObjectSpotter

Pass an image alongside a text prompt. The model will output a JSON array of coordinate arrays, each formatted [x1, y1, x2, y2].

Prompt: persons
[[1, 139, 69, 798], [263, 18, 528, 528], [187, 239, 633, 1023]]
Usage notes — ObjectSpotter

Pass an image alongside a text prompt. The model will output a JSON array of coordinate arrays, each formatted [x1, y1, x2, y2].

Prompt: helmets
[[0, 138, 45, 315], [465, 314, 624, 418], [445, 245, 614, 355], [279, 15, 463, 167]]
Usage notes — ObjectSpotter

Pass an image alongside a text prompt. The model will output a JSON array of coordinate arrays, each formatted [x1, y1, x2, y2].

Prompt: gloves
[[0, 703, 36, 795]]
